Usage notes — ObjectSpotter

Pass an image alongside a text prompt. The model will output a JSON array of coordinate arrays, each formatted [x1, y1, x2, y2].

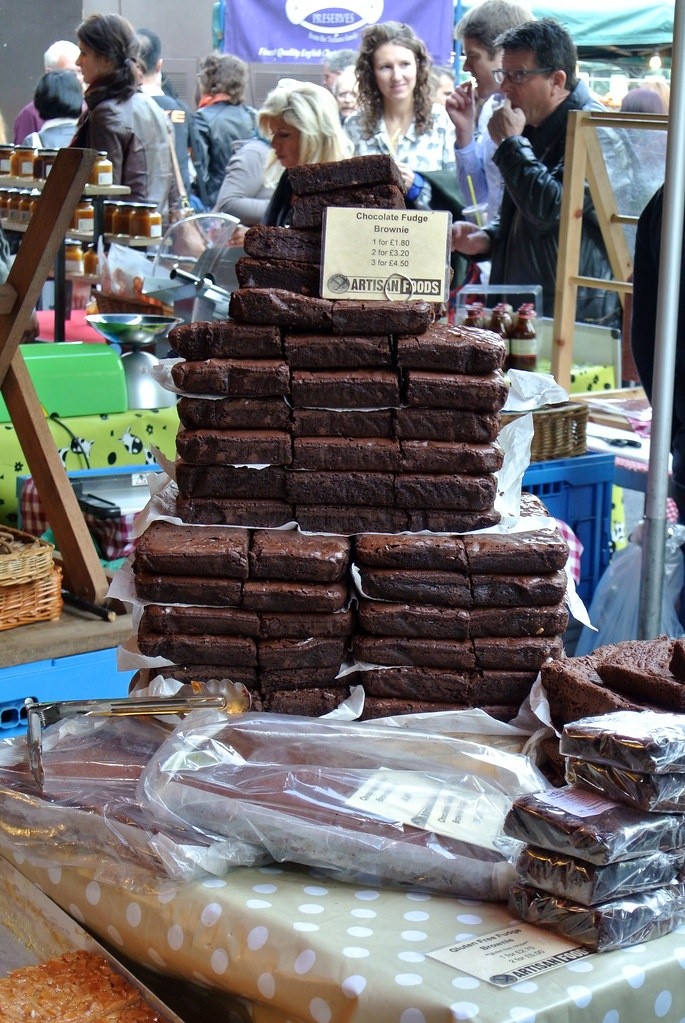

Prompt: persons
[[630, 185, 685, 637], [620, 78, 670, 182], [450, 19, 652, 330], [0, 232, 40, 344], [446, 0, 535, 286], [0, 14, 472, 287]]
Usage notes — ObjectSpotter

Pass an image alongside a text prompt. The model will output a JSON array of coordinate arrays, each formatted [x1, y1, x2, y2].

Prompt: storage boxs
[[521, 451, 626, 612]]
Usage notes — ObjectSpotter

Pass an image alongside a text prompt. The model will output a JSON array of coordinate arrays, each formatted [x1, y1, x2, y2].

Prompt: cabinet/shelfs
[[0, 176, 165, 286]]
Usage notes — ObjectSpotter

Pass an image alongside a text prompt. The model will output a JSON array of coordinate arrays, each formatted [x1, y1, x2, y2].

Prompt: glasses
[[489, 67, 566, 83]]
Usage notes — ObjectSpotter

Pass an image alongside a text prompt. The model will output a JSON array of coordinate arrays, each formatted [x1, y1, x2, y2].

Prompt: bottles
[[466, 303, 537, 373]]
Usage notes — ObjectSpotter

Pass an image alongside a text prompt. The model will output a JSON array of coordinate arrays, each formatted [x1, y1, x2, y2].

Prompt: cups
[[460, 204, 488, 228]]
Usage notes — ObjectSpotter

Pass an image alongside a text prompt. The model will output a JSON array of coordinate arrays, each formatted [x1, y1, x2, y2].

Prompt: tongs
[[24, 680, 252, 792]]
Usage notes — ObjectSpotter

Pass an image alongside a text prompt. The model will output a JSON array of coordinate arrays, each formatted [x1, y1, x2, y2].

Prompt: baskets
[[496, 402, 590, 462], [1, 523, 64, 631]]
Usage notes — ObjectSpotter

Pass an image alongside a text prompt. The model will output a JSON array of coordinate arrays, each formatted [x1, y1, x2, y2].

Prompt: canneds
[[0, 144, 162, 274]]
